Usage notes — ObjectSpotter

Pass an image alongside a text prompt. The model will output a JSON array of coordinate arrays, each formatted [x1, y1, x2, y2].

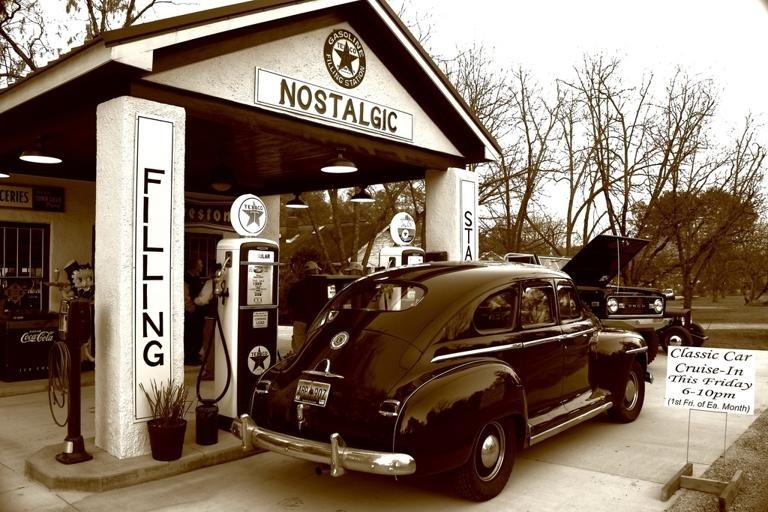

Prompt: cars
[[232, 261, 655, 505], [503, 233, 710, 356]]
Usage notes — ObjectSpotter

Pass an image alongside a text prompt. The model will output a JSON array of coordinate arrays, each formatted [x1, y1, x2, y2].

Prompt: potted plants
[[139, 376, 195, 463]]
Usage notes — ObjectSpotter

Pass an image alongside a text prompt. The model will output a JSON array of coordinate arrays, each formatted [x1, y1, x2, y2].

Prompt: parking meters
[[53, 297, 93, 464]]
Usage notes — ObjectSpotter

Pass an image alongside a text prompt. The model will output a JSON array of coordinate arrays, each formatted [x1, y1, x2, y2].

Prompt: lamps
[[349, 186, 376, 204], [285, 194, 310, 210], [16, 135, 64, 167], [320, 147, 359, 176]]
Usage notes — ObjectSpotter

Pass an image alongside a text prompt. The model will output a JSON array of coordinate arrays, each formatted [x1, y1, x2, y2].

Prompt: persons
[[189, 262, 219, 381], [182, 257, 205, 367], [344, 262, 365, 275], [288, 259, 323, 353]]
